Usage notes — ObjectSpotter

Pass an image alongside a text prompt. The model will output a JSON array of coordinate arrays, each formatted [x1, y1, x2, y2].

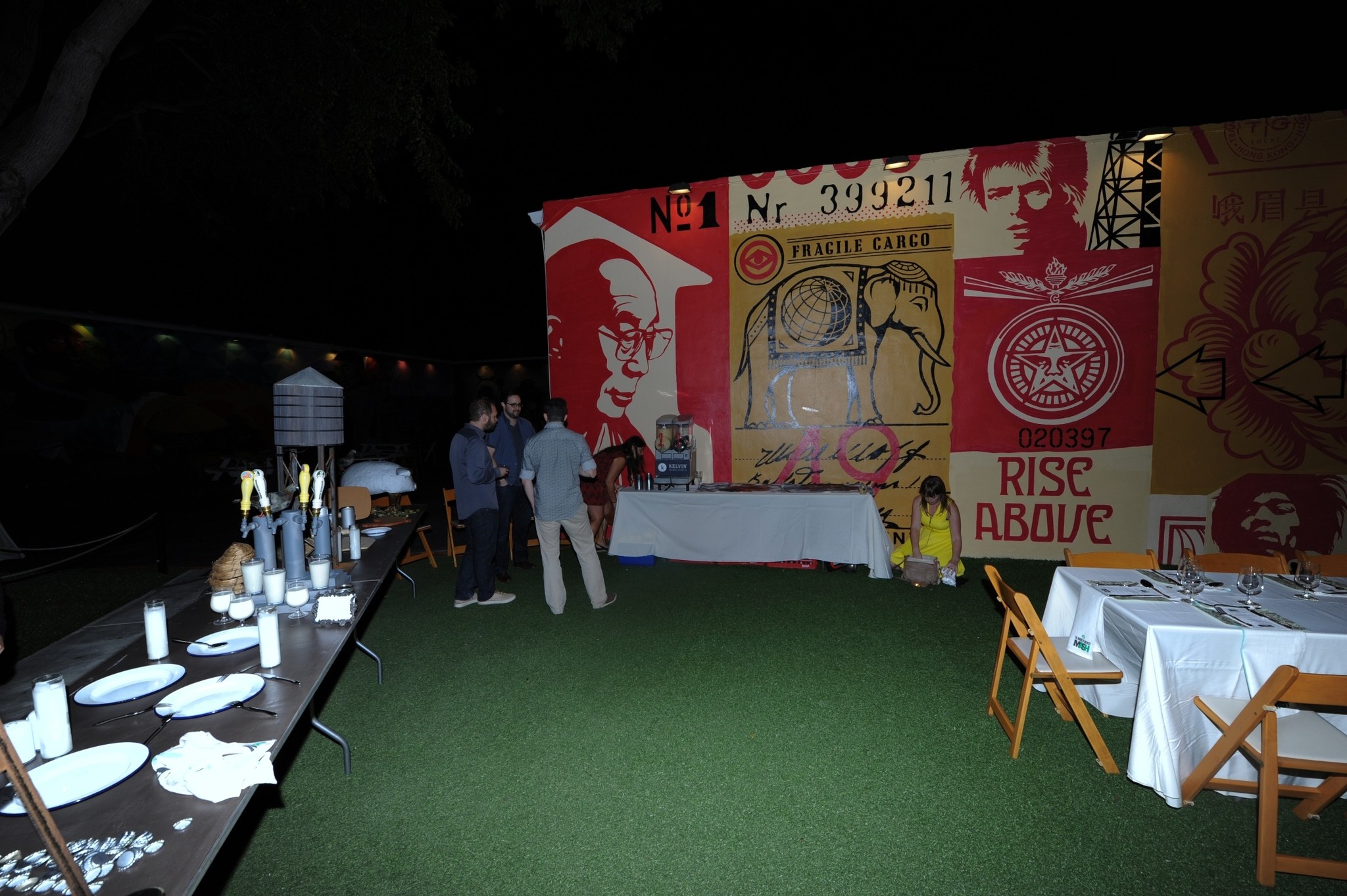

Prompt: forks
[[217, 660, 262, 684], [92, 703, 182, 747], [1095, 581, 1138, 587], [1215, 605, 1253, 627], [221, 701, 279, 717]]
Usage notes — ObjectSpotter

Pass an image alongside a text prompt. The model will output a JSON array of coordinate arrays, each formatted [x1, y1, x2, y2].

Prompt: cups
[[4, 669, 73, 767], [635, 472, 655, 491], [694, 470, 703, 486], [240, 553, 338, 669], [143, 597, 170, 662], [501, 464, 510, 469], [339, 505, 363, 560], [858, 484, 868, 494]]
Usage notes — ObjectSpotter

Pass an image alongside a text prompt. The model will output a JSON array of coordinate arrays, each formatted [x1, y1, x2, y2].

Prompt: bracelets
[[612, 501, 616, 503], [578, 435, 646, 554]]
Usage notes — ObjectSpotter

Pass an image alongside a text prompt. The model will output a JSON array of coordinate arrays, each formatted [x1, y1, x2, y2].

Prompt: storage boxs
[[618, 555, 818, 570]]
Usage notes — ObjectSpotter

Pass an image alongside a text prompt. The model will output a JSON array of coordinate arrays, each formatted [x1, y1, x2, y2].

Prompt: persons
[[519, 398, 617, 615], [449, 397, 516, 609], [483, 392, 537, 583], [889, 475, 965, 579]]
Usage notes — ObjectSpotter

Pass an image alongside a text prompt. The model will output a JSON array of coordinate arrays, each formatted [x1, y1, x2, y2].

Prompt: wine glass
[[1235, 566, 1265, 607], [1178, 557, 1205, 607], [209, 577, 312, 631], [1294, 561, 1322, 602]]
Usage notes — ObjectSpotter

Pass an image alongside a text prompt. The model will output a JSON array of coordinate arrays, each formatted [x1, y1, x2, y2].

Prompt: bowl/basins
[[841, 563, 856, 573]]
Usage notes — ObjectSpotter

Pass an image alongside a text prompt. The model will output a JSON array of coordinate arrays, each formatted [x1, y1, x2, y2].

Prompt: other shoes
[[515, 562, 536, 569], [594, 543, 608, 551], [495, 571, 512, 582]]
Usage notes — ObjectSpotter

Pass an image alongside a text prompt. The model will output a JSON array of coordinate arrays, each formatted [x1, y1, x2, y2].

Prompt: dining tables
[[1032, 566, 1347, 809], [0, 502, 428, 896], [606, 484, 896, 580]]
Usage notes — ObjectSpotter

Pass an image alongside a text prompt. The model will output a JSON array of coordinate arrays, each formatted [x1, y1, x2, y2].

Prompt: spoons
[[1140, 578, 1171, 598], [1205, 581, 1223, 587], [1321, 581, 1345, 590], [1195, 599, 1251, 609], [172, 638, 228, 649]]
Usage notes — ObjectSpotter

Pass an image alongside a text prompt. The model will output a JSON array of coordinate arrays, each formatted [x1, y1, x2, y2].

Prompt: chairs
[[1184, 547, 1291, 574], [1296, 553, 1347, 578], [1064, 548, 1161, 571], [984, 564, 1125, 774], [1179, 665, 1347, 887], [372, 488, 573, 580]]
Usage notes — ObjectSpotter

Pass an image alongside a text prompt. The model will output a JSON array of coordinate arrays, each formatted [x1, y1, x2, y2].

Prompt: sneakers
[[478, 591, 517, 604], [454, 593, 479, 607], [601, 592, 617, 607]]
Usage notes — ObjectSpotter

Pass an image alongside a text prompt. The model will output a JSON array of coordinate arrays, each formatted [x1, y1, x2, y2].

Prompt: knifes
[[1247, 608, 1293, 630], [1277, 573, 1316, 593], [1151, 568, 1181, 586], [255, 671, 303, 685]]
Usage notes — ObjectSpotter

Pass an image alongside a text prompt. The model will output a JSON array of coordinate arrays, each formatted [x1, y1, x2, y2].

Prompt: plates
[[361, 527, 392, 537], [74, 664, 186, 706], [154, 674, 267, 719], [186, 626, 260, 657], [0, 740, 151, 814]]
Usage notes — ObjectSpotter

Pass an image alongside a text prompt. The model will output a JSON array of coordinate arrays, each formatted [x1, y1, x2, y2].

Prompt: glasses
[[505, 402, 524, 407]]
